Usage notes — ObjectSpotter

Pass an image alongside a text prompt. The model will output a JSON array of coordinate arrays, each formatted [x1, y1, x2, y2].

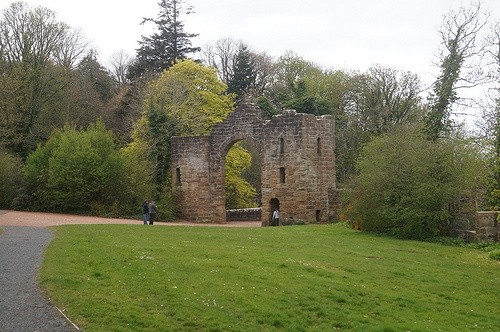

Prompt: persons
[[272, 208, 280, 226], [148, 201, 157, 225], [143, 201, 149, 224]]
